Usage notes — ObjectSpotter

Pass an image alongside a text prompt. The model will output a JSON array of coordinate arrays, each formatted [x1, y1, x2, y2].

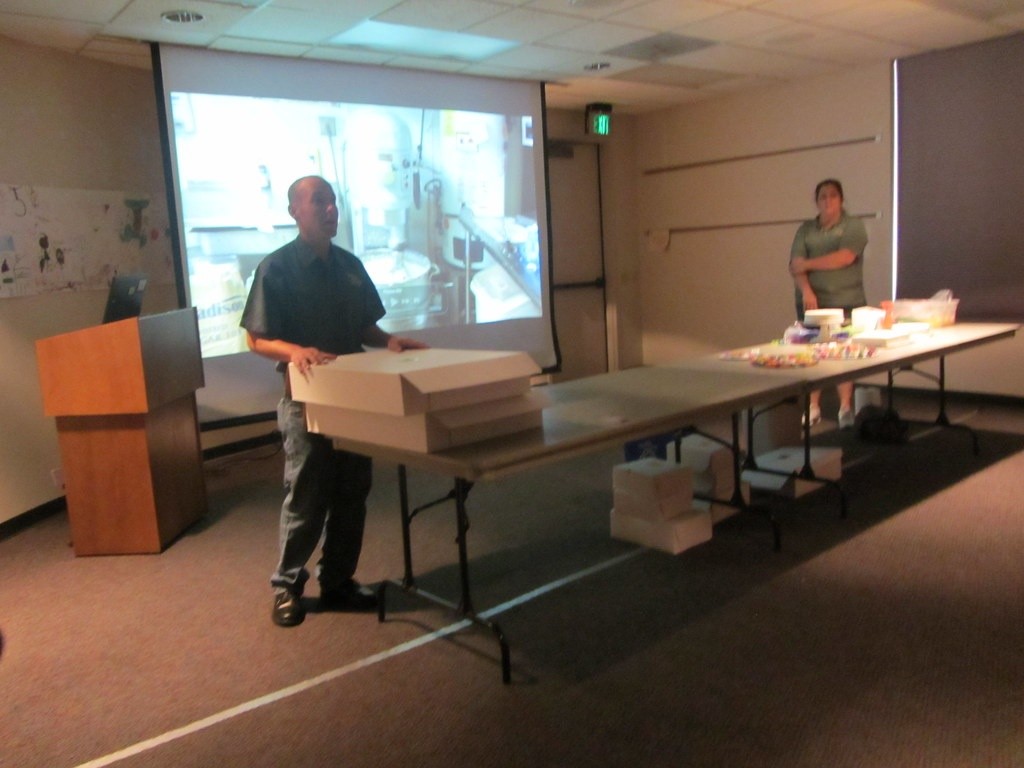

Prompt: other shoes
[[838, 410, 853, 430], [801, 407, 821, 427]]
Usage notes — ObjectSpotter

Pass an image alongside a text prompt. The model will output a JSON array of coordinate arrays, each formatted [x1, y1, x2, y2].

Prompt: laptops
[[102, 272, 149, 325]]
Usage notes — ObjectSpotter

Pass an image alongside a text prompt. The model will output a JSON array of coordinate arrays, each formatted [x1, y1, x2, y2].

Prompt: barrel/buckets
[[361, 246, 440, 332]]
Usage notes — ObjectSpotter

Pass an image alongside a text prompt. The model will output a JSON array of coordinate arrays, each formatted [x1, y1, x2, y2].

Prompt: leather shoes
[[272, 591, 306, 626], [320, 582, 379, 608]]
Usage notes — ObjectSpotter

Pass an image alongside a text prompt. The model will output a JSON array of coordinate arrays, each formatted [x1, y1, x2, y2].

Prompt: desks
[[328, 317, 1020, 687]]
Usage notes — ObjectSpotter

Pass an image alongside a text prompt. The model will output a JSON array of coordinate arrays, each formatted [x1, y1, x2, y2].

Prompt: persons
[[239, 175, 433, 631], [787, 178, 870, 428]]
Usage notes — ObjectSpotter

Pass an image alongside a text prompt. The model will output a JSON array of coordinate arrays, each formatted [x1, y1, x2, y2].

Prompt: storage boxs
[[609, 388, 842, 557], [288, 345, 545, 454]]
